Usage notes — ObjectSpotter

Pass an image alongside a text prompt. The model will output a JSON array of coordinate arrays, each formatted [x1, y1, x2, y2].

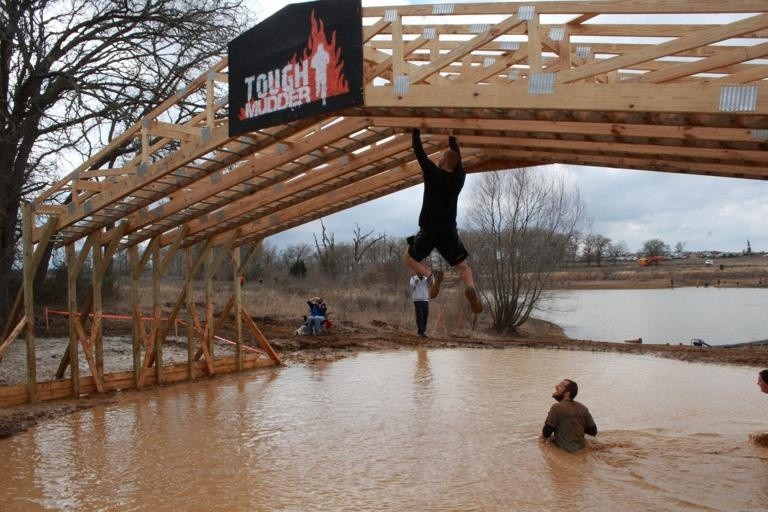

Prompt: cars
[[618, 252, 744, 266]]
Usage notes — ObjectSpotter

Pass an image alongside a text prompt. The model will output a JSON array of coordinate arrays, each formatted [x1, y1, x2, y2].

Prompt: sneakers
[[427, 270, 444, 299], [465, 286, 483, 313]]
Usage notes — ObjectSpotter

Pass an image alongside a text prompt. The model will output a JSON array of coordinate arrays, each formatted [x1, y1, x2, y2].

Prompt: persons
[[305, 296, 326, 337], [758, 369, 768, 394], [541, 378, 596, 453], [258, 275, 263, 282], [410, 270, 430, 338], [240, 274, 246, 286], [405, 128, 483, 313]]
